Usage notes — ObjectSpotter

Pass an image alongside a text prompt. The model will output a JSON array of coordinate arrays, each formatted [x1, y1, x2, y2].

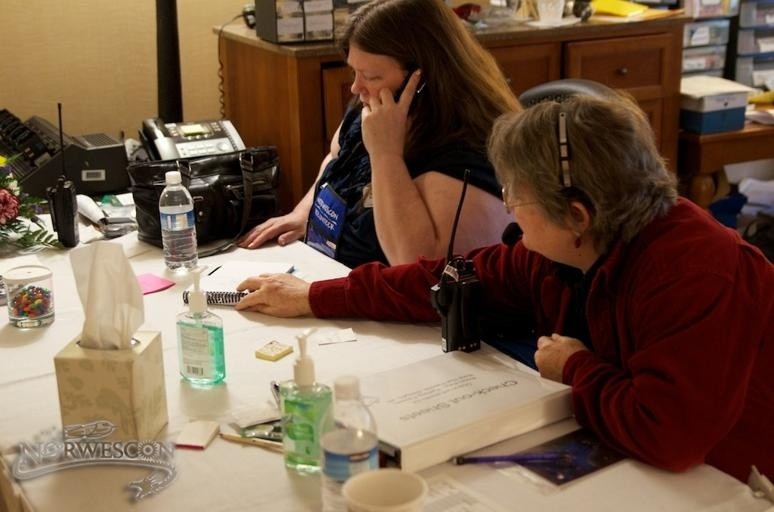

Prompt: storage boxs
[[51, 325, 173, 455], [675, 75, 750, 139]]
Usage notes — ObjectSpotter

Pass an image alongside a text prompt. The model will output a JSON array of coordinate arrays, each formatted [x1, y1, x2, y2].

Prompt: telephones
[[138, 117, 247, 164]]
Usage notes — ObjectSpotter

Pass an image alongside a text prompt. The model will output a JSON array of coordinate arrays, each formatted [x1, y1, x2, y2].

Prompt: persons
[[236, 0, 524, 269], [234, 89, 773, 508]]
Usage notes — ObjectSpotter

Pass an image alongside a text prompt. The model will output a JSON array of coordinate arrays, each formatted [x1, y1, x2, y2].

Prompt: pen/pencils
[[219, 432, 285, 450], [453, 452, 571, 466]]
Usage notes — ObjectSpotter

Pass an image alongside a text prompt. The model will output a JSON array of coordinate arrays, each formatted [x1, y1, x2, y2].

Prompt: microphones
[[502, 221, 522, 245]]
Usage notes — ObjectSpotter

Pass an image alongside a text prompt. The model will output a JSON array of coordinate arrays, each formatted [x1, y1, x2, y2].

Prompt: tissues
[[52, 240, 170, 445]]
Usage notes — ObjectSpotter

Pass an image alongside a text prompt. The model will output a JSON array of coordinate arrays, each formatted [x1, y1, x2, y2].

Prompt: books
[[182, 260, 296, 308]]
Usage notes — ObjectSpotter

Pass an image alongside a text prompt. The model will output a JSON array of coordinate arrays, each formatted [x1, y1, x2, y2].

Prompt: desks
[[0, 199, 772, 512], [681, 112, 774, 212]]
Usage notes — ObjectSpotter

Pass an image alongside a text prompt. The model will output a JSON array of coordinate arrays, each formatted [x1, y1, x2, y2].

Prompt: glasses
[[499, 186, 541, 214]]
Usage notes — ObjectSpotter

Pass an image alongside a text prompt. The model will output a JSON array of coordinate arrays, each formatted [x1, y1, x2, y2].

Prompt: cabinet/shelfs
[[215, 10, 682, 225]]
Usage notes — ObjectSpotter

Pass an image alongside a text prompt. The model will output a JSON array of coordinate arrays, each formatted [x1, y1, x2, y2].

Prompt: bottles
[[159, 170, 199, 270], [318, 373, 380, 510]]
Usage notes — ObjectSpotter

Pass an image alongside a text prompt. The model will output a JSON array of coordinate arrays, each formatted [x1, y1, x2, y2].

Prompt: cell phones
[[393, 60, 426, 117]]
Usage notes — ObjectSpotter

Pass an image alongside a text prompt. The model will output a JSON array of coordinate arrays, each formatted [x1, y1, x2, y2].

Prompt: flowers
[[0, 155, 65, 253]]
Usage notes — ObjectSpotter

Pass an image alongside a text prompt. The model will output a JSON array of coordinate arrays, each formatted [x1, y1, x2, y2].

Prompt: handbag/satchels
[[126, 146, 272, 251]]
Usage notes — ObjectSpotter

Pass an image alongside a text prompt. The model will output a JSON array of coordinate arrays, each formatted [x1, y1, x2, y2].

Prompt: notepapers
[[255, 339, 293, 361], [174, 420, 220, 450]]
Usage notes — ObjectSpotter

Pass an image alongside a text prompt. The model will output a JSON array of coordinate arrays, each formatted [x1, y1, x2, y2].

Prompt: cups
[[338, 466, 430, 511], [2, 263, 56, 329], [537, 1, 564, 22]]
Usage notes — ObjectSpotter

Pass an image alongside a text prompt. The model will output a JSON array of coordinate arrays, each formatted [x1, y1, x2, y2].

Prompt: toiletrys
[[175, 265, 227, 385], [277, 326, 334, 478]]
